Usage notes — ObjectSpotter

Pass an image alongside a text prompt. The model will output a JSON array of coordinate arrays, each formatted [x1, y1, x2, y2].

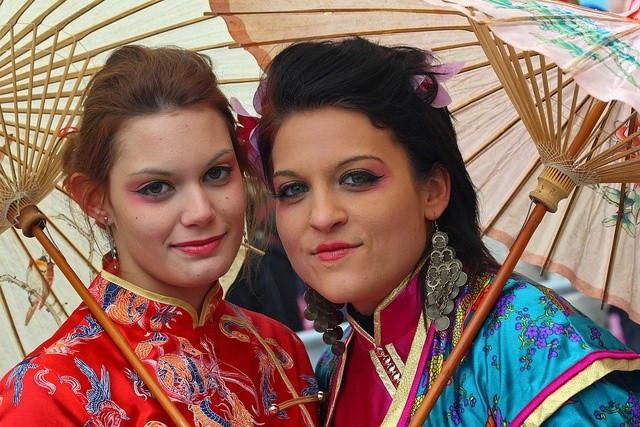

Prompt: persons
[[1, 45, 323, 427], [255, 34, 637, 427]]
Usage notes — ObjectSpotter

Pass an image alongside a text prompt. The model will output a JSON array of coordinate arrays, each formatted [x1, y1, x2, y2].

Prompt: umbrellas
[[209, 2, 640, 427], [1, 2, 265, 427]]
